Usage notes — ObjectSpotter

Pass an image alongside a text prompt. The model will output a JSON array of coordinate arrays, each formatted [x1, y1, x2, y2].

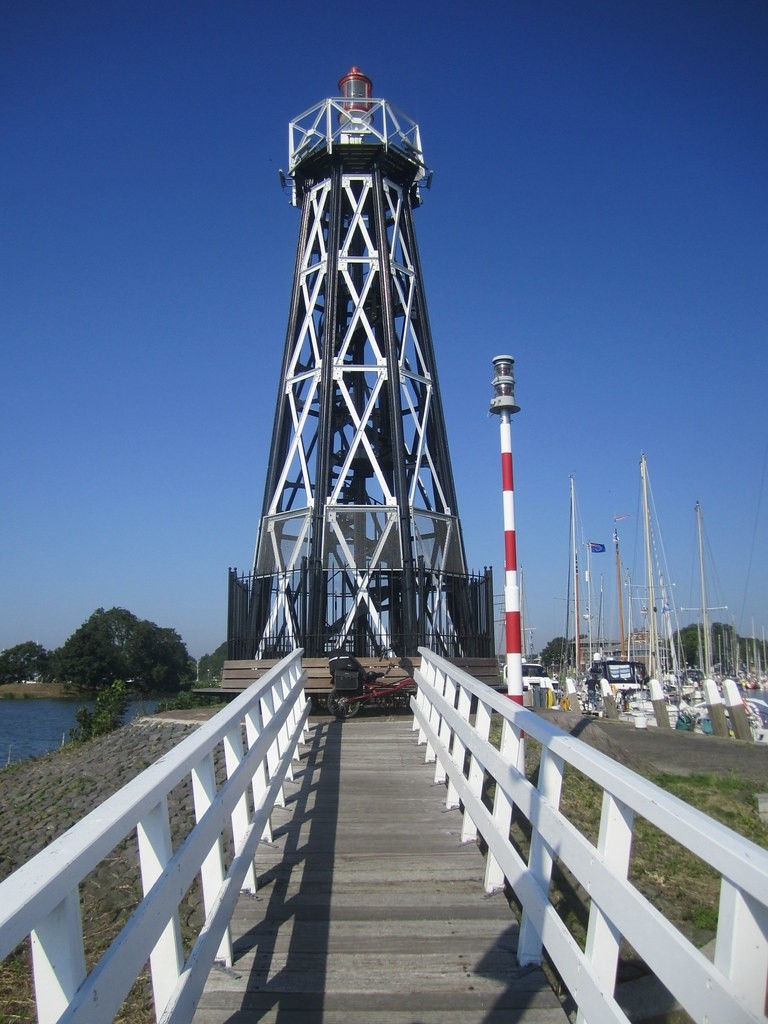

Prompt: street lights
[[490, 355, 525, 777]]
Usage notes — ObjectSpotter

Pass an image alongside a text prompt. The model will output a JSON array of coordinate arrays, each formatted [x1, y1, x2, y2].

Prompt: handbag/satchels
[[335, 669, 360, 690], [329, 649, 364, 674]]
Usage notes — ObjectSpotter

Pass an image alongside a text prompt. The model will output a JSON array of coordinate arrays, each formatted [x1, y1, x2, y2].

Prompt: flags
[[591, 543, 606, 553]]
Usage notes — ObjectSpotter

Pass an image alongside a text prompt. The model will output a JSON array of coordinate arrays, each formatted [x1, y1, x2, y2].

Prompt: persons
[[637, 671, 644, 690], [585, 675, 600, 714], [591, 665, 599, 681]]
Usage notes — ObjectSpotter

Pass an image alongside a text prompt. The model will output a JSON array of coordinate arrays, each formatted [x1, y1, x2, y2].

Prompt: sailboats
[[569, 453, 768, 744]]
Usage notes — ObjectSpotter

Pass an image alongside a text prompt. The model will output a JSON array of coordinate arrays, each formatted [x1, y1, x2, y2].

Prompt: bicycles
[[328, 670, 414, 718]]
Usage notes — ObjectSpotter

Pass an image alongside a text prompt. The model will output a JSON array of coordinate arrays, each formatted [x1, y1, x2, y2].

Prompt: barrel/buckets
[[635, 717, 649, 728]]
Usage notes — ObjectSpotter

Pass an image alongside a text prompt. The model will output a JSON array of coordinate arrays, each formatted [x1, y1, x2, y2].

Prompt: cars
[[503, 664, 563, 698]]
